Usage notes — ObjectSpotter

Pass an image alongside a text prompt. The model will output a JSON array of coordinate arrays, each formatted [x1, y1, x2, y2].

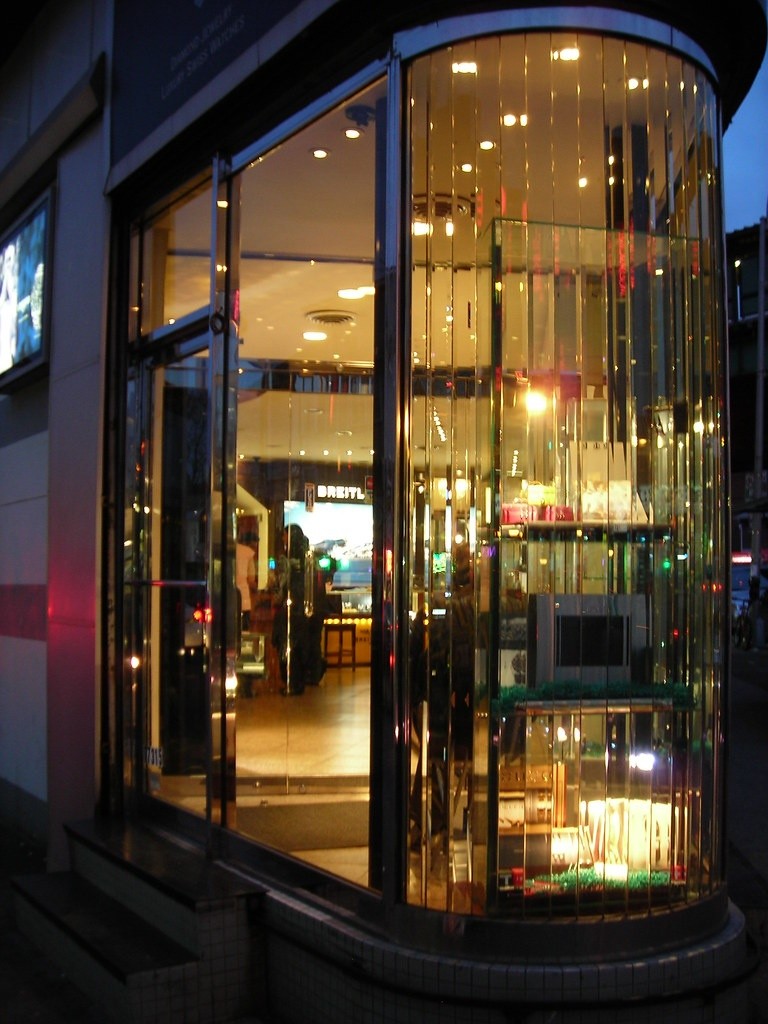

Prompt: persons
[[408, 541, 528, 852], [233, 523, 257, 698], [239, 528, 260, 580], [271, 524, 308, 697], [302, 537, 329, 684]]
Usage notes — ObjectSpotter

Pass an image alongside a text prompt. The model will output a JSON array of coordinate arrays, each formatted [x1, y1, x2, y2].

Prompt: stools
[[326, 625, 356, 671]]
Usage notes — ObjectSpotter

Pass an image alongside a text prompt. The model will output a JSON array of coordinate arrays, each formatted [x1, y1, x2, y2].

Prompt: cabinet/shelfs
[[320, 591, 371, 666], [476, 526, 693, 911], [250, 589, 282, 695]]
